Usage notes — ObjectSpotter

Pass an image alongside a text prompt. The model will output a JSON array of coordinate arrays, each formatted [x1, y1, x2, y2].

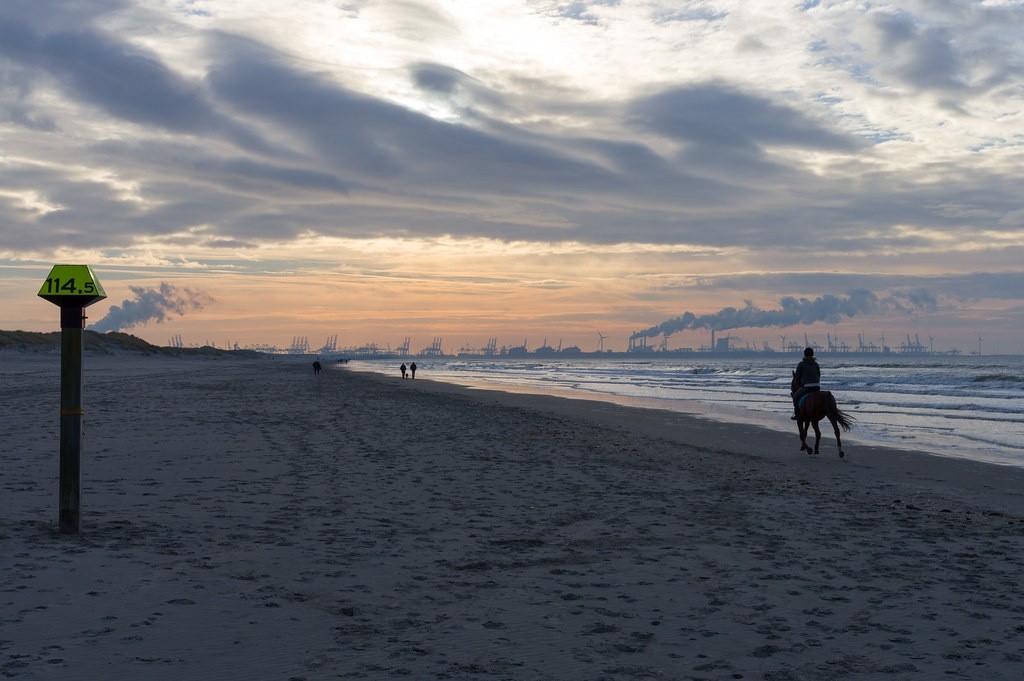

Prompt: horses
[[791, 370, 856, 458]]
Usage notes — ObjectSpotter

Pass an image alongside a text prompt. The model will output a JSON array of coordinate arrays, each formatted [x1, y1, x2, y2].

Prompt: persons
[[312, 360, 321, 375], [410, 362, 416, 379], [790, 347, 821, 420], [400, 363, 406, 379]]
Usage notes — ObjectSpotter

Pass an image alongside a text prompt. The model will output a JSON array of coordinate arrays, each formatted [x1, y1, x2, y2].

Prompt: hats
[[804, 347, 814, 357]]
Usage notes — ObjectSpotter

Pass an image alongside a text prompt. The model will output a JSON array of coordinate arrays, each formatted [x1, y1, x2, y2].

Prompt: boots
[[791, 405, 800, 420]]
